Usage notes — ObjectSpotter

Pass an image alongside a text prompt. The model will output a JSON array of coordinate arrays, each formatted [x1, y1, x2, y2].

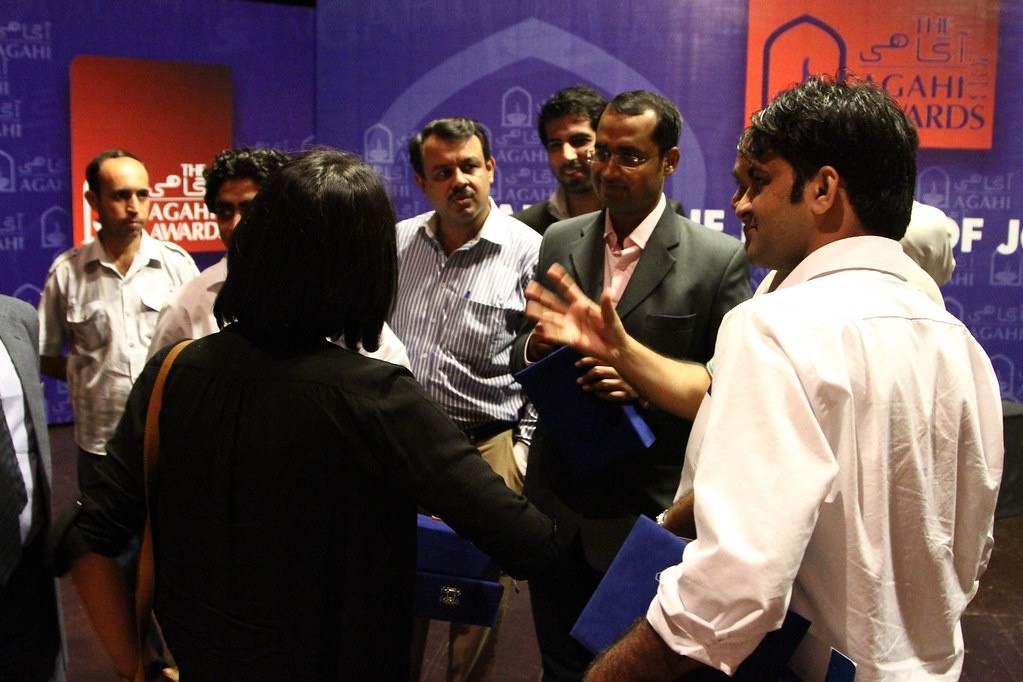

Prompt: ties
[[0, 401, 28, 515]]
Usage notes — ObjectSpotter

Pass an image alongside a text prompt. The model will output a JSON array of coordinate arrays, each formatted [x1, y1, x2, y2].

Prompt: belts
[[468, 420, 516, 446]]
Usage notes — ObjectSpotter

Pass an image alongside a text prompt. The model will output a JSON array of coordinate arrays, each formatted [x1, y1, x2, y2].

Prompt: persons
[[37, 151, 203, 492], [149, 145, 413, 369], [49, 149, 577, 681], [508, 90, 748, 682], [0, 294, 71, 682], [383, 119, 544, 682], [524, 71, 1004, 681], [510, 84, 685, 235]]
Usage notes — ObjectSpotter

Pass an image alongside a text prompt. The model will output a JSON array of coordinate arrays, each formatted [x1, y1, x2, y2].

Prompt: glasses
[[585, 145, 659, 174]]
